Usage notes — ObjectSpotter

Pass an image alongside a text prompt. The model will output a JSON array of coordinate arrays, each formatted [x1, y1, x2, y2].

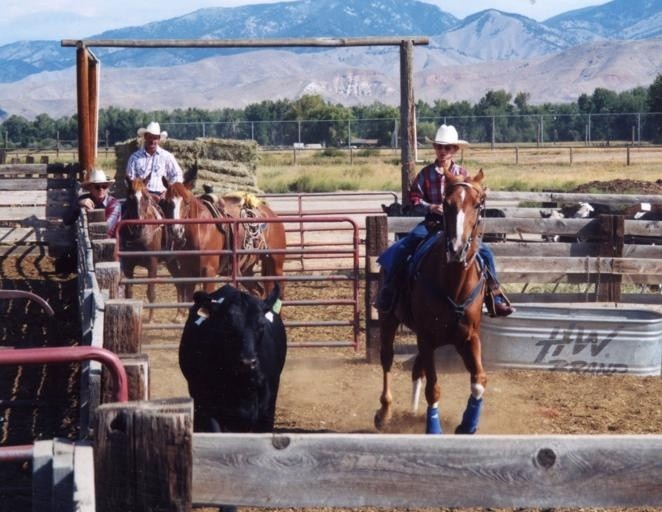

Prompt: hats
[[429, 123, 471, 149], [136, 120, 169, 141], [82, 169, 117, 187]]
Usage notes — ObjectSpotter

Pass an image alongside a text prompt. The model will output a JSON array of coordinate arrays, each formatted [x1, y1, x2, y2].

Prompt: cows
[[381, 191, 412, 241], [593, 203, 662, 246], [178, 281, 287, 512], [480, 209, 506, 242], [539, 202, 611, 243]]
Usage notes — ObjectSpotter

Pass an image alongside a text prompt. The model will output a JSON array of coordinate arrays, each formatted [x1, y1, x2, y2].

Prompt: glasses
[[436, 145, 455, 151], [93, 185, 109, 191]]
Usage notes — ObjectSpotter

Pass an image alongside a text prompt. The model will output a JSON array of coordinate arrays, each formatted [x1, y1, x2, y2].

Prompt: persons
[[372, 123, 516, 318], [70, 166, 123, 299], [122, 122, 186, 249]]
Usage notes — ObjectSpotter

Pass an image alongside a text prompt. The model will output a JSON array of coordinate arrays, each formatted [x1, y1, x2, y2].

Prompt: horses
[[118, 171, 169, 323], [368, 167, 488, 434], [162, 176, 287, 324]]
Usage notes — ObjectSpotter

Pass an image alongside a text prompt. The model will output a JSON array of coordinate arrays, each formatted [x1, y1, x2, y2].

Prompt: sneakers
[[377, 286, 395, 314], [487, 301, 516, 318]]
